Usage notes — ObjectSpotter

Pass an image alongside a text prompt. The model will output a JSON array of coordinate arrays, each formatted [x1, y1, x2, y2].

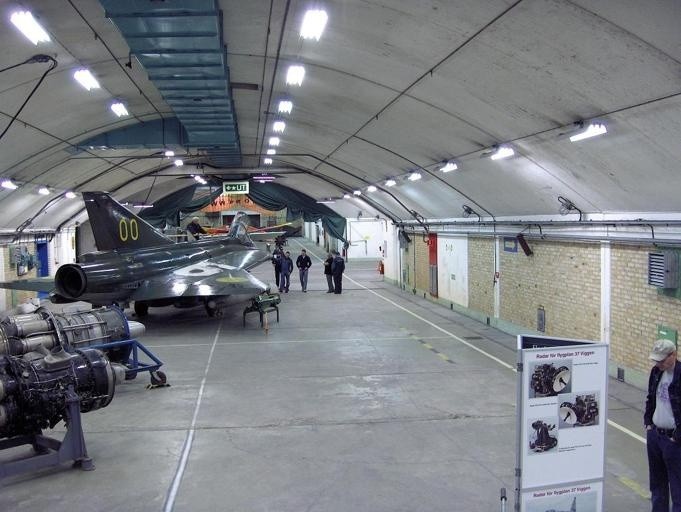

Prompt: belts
[[652, 425, 673, 436]]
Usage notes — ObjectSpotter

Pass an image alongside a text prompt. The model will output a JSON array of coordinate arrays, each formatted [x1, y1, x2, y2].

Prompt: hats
[[648, 338, 677, 362]]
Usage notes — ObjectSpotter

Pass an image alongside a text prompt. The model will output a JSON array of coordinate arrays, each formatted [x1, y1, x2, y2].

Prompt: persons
[[296, 250, 312, 293], [324, 254, 335, 293], [643, 338, 681, 512], [184, 217, 207, 241], [271, 234, 293, 293], [331, 252, 345, 294]]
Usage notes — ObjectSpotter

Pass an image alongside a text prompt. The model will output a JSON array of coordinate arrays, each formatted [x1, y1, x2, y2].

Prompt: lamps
[[373, 214, 388, 232], [462, 205, 480, 223], [557, 196, 581, 222], [15, 217, 34, 233], [263, 8, 329, 166], [343, 144, 517, 200], [407, 210, 424, 225], [569, 119, 610, 143], [9, 2, 130, 119]]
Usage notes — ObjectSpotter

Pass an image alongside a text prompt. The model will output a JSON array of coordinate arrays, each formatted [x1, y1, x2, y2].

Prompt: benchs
[[242, 305, 279, 328]]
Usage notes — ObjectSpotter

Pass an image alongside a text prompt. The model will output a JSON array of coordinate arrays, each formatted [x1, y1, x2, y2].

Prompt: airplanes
[[1, 188, 292, 321]]
